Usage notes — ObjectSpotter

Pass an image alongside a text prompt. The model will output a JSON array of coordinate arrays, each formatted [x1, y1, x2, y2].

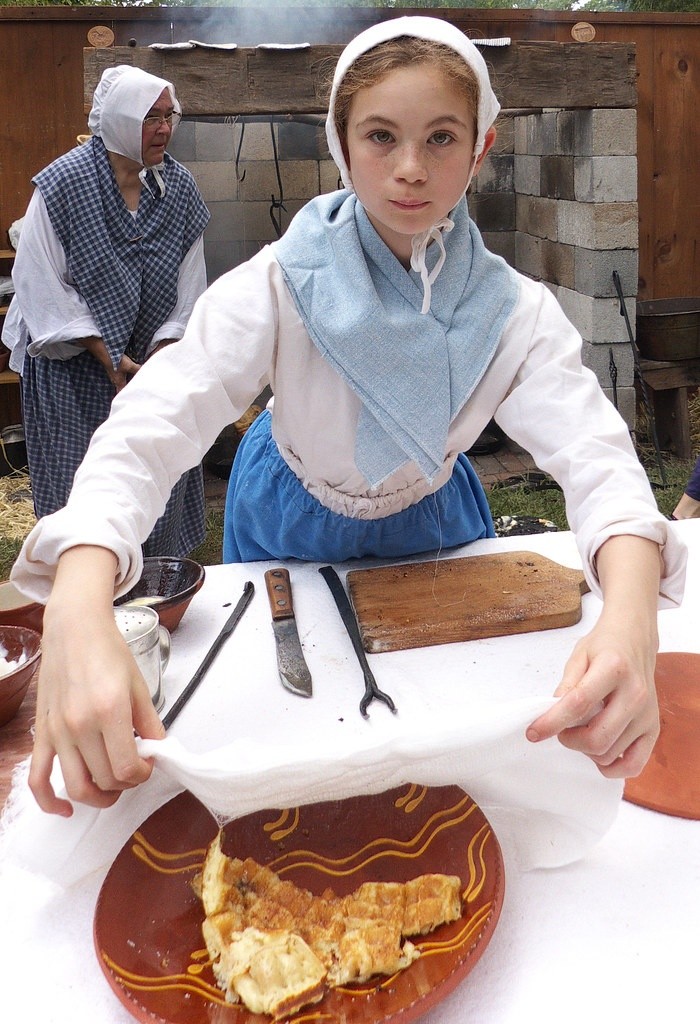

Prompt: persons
[[2, 65, 212, 556], [9, 16, 690, 818], [667, 460, 700, 521]]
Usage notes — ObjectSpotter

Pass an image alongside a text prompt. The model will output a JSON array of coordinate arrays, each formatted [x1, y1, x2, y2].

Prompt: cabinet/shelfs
[[0, 248, 20, 384]]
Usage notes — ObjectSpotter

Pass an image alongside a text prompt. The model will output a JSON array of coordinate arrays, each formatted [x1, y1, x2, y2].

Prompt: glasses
[[143, 111, 182, 129]]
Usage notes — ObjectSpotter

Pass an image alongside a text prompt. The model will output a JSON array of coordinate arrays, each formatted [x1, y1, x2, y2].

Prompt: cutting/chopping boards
[[347, 550, 603, 653]]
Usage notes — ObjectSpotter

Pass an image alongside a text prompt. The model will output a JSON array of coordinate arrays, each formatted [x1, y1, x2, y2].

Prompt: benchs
[[634, 351, 700, 459]]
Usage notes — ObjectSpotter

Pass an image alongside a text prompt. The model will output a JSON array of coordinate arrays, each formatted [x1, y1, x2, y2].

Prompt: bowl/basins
[[637, 293, 700, 360], [113, 551, 207, 633], [0, 621, 41, 728], [0, 578, 45, 630]]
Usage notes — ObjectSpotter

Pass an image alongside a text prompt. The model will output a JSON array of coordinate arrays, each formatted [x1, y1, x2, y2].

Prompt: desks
[[0, 517, 700, 1024]]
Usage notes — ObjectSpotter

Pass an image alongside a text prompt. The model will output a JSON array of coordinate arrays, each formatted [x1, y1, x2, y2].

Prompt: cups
[[113, 606, 171, 713]]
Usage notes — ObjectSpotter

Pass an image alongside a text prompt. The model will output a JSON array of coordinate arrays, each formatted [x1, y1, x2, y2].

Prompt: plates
[[93, 773, 507, 1022]]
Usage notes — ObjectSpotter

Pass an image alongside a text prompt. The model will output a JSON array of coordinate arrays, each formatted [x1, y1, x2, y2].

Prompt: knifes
[[263, 566, 315, 700]]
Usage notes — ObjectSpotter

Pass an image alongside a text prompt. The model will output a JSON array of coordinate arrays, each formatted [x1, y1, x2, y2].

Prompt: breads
[[197, 847, 459, 1021]]
